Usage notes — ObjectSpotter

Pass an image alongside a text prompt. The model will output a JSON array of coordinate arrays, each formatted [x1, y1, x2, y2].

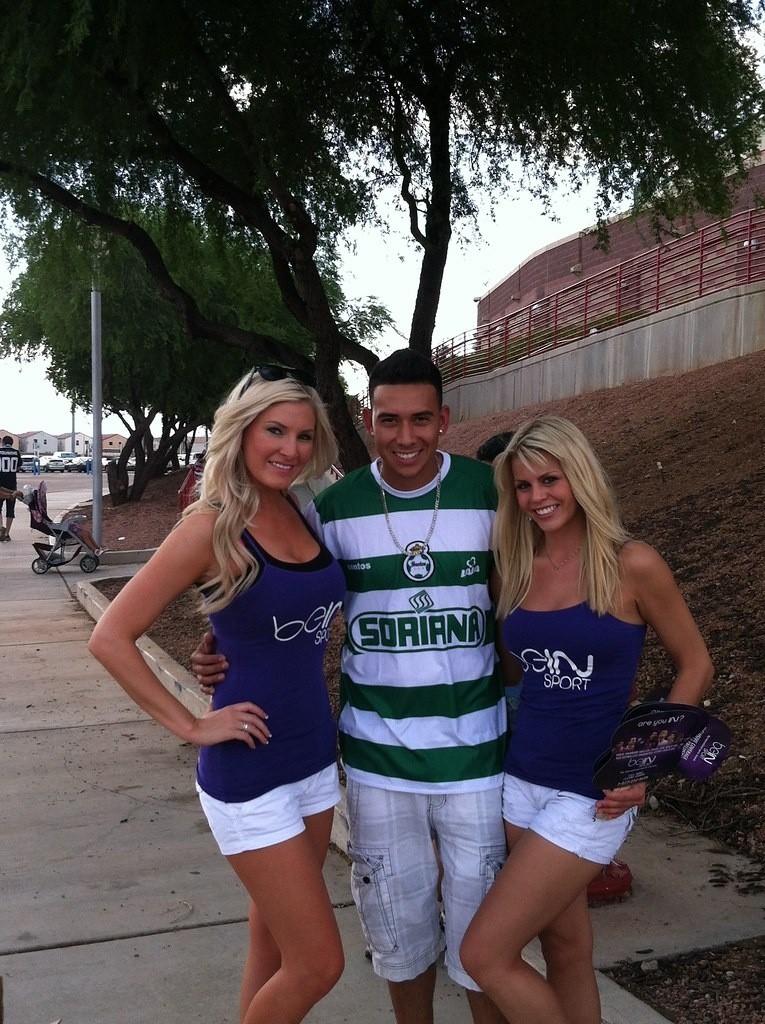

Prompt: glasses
[[238, 364, 320, 400]]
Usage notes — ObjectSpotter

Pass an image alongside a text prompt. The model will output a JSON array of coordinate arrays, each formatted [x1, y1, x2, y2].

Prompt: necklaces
[[377, 451, 444, 560], [535, 532, 588, 570]]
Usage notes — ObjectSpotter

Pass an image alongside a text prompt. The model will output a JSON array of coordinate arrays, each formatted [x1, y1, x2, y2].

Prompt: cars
[[21, 450, 204, 474]]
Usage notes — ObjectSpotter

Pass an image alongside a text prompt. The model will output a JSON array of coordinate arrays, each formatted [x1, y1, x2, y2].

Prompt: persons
[[86, 360, 351, 1023], [459, 415, 717, 1024], [0, 434, 23, 543], [186, 345, 540, 1024]]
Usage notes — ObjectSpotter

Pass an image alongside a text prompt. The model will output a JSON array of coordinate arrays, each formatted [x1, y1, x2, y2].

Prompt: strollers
[[14, 480, 99, 574]]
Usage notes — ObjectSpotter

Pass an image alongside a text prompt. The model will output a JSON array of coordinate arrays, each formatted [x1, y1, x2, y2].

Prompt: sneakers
[[7, 534, 13, 541], [0, 528, 6, 543]]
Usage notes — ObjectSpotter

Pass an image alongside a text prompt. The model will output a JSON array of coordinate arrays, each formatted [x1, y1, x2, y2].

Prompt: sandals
[[93, 547, 108, 557]]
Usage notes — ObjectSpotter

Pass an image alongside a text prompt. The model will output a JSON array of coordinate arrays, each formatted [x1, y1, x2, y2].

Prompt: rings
[[241, 721, 249, 733]]
